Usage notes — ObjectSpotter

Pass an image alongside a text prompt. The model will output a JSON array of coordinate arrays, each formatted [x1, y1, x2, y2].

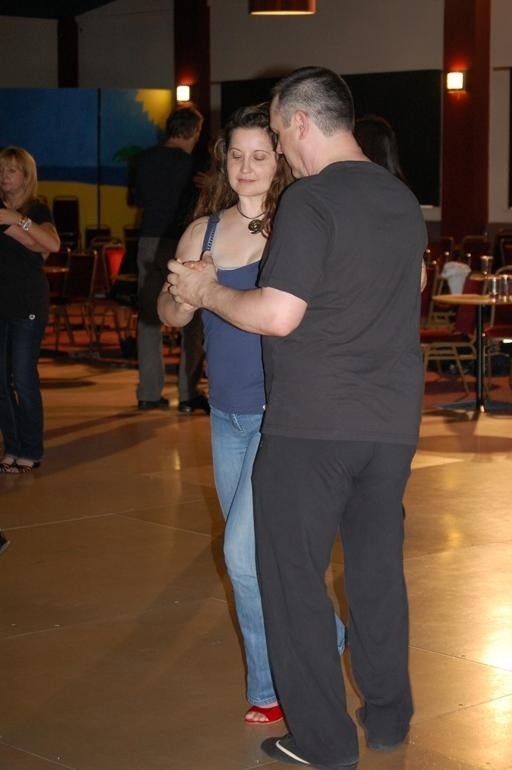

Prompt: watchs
[[23, 217, 31, 232]]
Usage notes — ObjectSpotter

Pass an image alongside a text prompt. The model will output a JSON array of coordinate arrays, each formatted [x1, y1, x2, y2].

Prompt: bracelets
[[16, 214, 27, 227]]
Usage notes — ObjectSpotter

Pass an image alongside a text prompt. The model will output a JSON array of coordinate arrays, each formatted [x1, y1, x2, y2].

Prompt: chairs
[[43, 195, 181, 354], [420, 235, 512, 412]]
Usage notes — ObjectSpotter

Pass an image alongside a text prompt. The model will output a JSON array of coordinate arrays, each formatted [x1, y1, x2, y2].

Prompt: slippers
[[140, 398, 169, 408], [7, 459, 41, 474], [180, 395, 210, 413], [244, 705, 284, 724], [0, 461, 14, 471], [261, 735, 358, 768]]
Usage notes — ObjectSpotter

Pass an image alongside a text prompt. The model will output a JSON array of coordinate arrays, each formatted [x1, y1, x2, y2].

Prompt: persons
[[125, 107, 210, 415], [353, 114, 428, 294], [0, 146, 60, 474], [167, 63, 423, 770], [193, 128, 227, 192], [155, 99, 346, 728], [0, 197, 49, 261]]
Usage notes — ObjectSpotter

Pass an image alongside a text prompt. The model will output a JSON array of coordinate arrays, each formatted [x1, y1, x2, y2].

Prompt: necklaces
[[236, 201, 267, 235]]
